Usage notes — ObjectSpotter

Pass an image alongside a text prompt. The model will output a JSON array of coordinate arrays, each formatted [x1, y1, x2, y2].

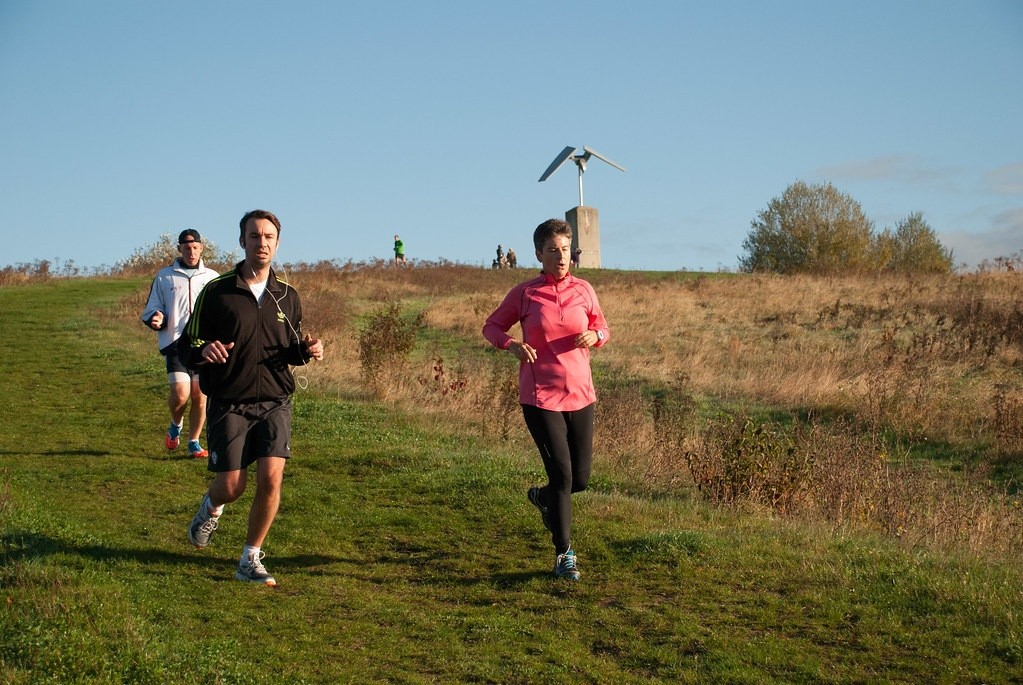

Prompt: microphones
[[251, 268, 257, 277]]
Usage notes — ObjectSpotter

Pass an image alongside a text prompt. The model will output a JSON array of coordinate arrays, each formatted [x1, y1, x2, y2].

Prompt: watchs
[[590, 327, 604, 347]]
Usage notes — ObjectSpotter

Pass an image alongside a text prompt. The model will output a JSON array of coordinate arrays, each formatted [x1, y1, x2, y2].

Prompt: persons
[[490, 243, 518, 268], [482, 217, 608, 585], [141, 230, 221, 462], [392, 235, 407, 267], [177, 209, 325, 586], [572, 246, 582, 268]]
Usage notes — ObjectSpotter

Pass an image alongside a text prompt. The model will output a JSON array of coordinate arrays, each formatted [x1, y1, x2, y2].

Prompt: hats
[[178, 229, 201, 244]]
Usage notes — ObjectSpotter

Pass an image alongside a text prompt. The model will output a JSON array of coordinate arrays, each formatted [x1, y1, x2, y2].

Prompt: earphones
[[242, 242, 245, 249], [276, 242, 280, 251]]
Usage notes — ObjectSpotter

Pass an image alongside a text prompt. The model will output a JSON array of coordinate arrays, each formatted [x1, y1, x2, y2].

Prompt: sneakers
[[238, 556, 276, 586], [189, 493, 224, 547], [527, 486, 552, 531], [550, 549, 581, 581], [188, 441, 209, 458], [164, 416, 184, 449]]
